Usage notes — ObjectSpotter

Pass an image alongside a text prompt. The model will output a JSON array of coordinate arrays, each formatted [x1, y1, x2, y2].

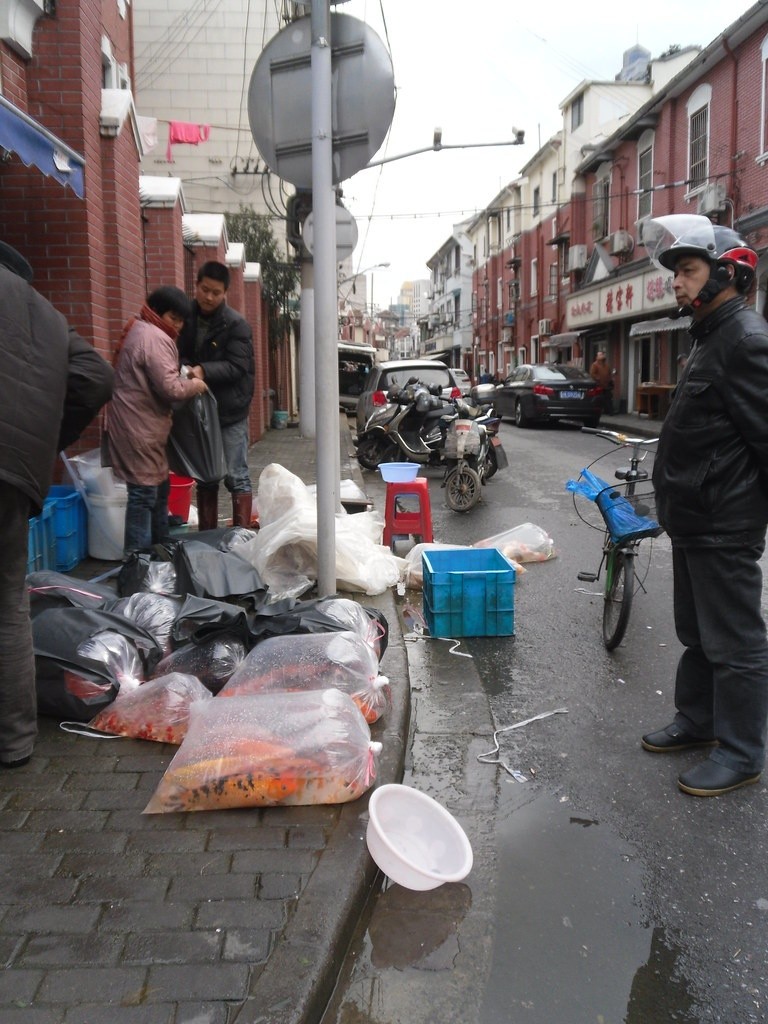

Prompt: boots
[[196, 490, 219, 530], [232, 493, 260, 530]]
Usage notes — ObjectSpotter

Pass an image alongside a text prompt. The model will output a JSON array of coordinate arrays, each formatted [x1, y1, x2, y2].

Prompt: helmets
[[658, 224, 759, 293]]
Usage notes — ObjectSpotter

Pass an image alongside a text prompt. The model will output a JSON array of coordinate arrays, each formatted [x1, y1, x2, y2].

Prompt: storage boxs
[[470, 382, 498, 405], [422, 547, 515, 639], [27, 485, 87, 603]]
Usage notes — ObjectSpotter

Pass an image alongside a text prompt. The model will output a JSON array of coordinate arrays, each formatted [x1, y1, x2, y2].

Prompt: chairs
[[339, 362, 349, 385], [356, 365, 368, 383]]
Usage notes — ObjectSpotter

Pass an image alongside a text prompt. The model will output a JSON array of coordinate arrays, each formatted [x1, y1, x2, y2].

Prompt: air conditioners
[[539, 319, 551, 336], [501, 326, 512, 343], [568, 244, 587, 272], [636, 216, 658, 246], [696, 183, 728, 218], [609, 230, 629, 257]]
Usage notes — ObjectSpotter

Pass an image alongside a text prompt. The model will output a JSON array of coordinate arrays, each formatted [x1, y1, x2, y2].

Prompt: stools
[[383, 477, 432, 548]]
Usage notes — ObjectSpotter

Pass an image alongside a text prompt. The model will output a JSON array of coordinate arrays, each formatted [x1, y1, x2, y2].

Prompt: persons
[[105, 285, 207, 560], [677, 353, 689, 370], [178, 259, 256, 532], [642, 225, 768, 796], [0, 235, 119, 769], [481, 368, 493, 385], [590, 351, 615, 416]]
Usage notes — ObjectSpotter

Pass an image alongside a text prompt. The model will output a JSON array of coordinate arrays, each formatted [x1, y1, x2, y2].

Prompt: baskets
[[594, 479, 666, 546]]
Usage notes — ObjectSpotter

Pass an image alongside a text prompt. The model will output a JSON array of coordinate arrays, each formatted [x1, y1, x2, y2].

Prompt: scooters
[[347, 376, 509, 479]]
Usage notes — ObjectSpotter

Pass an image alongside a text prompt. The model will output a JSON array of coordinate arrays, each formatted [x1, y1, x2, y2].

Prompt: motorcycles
[[439, 396, 500, 513]]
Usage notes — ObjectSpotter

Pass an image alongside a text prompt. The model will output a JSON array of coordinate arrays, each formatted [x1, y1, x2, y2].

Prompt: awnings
[[0, 93, 85, 201], [420, 346, 457, 360], [629, 314, 693, 336], [549, 329, 588, 344]]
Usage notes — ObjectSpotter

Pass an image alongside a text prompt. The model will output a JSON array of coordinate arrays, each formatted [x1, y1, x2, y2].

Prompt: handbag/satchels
[[565, 467, 661, 541]]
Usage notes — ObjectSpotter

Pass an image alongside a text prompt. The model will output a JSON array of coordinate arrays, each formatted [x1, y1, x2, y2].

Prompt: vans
[[337, 340, 376, 414]]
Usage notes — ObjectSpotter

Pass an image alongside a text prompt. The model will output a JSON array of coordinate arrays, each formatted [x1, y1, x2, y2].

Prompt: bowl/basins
[[365, 783, 473, 891], [378, 462, 422, 483]]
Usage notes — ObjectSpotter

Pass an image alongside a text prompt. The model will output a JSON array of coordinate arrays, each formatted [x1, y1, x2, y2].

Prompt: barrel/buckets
[[167, 474, 195, 523], [88, 493, 128, 560], [274, 412, 288, 429]]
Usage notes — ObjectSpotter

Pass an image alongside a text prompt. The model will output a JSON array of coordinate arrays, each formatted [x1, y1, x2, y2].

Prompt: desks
[[636, 383, 677, 421]]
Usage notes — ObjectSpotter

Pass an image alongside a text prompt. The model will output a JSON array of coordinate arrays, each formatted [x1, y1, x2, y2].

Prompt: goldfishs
[[95, 665, 380, 812]]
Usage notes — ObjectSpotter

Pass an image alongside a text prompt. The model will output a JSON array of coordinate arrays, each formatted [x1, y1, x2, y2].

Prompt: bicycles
[[577, 426, 660, 650]]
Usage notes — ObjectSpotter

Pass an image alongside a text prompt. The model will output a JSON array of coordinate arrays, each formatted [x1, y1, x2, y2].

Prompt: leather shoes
[[678, 758, 762, 797], [641, 723, 717, 752]]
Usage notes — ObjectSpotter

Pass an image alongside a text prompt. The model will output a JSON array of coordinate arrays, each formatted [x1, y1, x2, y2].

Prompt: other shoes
[[0, 756, 30, 767]]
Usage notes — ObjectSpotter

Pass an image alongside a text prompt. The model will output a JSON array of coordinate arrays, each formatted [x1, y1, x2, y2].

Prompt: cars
[[348, 360, 463, 438], [450, 368, 472, 396], [491, 363, 603, 427]]
